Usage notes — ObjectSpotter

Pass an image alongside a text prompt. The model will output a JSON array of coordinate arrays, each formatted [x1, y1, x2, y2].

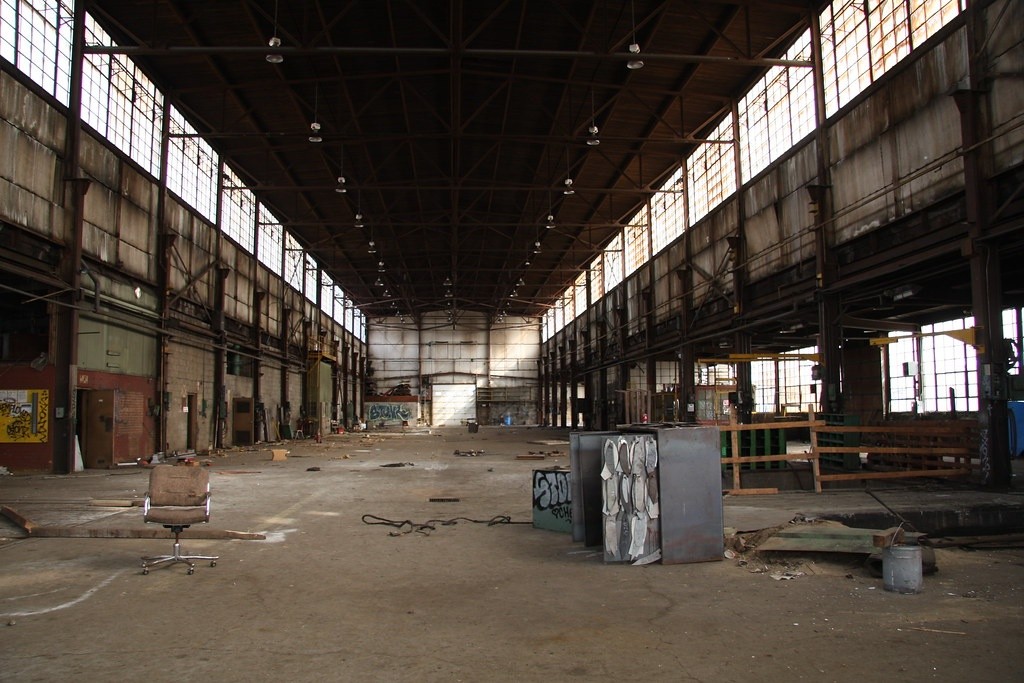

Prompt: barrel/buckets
[[883, 520, 922, 594], [505, 416, 510, 425]]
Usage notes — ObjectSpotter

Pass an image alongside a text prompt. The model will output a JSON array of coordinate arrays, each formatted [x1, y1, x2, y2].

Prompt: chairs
[[141, 465, 219, 575]]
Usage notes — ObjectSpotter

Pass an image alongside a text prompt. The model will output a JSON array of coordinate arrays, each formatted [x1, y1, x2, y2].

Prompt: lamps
[[444, 289, 453, 298], [308, 86, 323, 143], [545, 193, 556, 228], [374, 276, 384, 287], [354, 189, 365, 228], [563, 148, 575, 195], [377, 243, 386, 273], [368, 222, 377, 254], [509, 290, 519, 298], [265, 0, 283, 63], [626, 0, 645, 69], [515, 278, 525, 286], [533, 225, 544, 254], [586, 89, 601, 146], [442, 276, 453, 286], [382, 289, 391, 297], [334, 141, 347, 193]]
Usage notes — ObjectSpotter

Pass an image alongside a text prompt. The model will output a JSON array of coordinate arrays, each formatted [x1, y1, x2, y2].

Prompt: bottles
[[642, 414, 647, 423]]
